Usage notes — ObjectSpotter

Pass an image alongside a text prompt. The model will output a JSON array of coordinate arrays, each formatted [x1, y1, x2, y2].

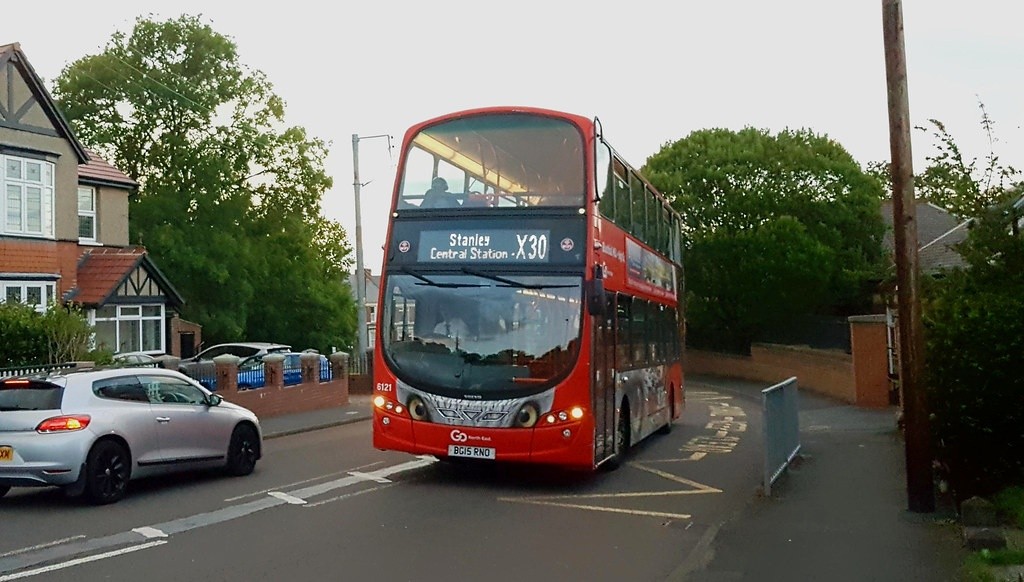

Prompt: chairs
[[462, 191, 487, 208]]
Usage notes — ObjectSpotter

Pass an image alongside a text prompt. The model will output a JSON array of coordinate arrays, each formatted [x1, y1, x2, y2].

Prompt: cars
[[0, 366, 264, 507], [107, 353, 159, 368], [199, 353, 333, 392]]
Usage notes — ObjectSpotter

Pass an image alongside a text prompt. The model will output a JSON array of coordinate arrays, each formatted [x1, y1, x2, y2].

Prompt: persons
[[425, 177, 448, 195], [433, 301, 470, 340]]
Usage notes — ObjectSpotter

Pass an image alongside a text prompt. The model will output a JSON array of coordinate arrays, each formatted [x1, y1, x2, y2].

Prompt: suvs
[[178, 342, 292, 382]]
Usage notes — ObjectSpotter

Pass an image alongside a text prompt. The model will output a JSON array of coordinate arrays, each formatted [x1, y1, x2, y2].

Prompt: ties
[[444, 324, 451, 337]]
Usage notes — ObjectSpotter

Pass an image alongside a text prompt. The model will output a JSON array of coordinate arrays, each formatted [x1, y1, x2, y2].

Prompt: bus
[[371, 104, 684, 476]]
